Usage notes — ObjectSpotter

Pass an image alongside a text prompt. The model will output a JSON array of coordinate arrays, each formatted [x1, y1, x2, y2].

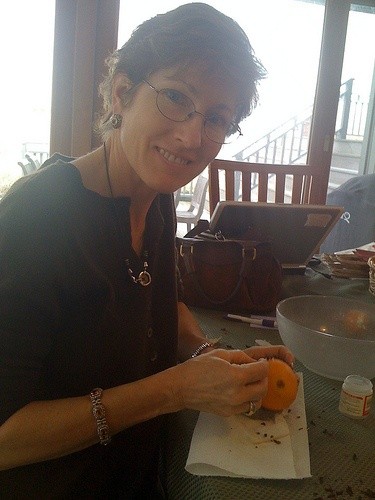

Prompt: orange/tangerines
[[260, 358, 298, 412]]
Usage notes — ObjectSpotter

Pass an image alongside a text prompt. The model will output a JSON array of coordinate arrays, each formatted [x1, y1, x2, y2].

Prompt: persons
[[320, 174, 375, 258], [1, 2, 296, 500]]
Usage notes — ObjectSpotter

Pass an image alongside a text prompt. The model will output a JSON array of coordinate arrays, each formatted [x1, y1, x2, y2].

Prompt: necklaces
[[104, 137, 151, 286]]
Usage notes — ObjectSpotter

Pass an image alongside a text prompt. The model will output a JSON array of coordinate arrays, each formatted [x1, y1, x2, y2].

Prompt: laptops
[[210, 201, 344, 274]]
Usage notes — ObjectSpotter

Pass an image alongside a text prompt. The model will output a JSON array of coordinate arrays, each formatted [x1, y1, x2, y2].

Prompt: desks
[[162, 267, 375, 500]]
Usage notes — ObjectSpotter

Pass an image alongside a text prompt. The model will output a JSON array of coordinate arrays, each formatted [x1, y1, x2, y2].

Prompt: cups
[[339, 375, 374, 420]]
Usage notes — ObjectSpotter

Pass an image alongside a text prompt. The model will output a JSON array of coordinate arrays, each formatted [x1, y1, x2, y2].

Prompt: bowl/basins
[[276, 295, 375, 383]]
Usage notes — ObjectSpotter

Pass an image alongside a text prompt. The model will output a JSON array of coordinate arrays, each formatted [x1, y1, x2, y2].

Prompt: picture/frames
[[208, 200, 344, 266]]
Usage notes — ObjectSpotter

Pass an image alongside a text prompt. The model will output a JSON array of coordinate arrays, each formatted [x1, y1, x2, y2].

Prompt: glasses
[[137, 71, 244, 145]]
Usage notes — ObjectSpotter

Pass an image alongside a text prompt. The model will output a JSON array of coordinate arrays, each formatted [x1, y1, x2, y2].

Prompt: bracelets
[[89, 387, 113, 446], [190, 342, 212, 357]]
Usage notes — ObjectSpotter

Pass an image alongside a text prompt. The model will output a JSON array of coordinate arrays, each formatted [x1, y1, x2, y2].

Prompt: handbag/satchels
[[175, 218, 270, 313]]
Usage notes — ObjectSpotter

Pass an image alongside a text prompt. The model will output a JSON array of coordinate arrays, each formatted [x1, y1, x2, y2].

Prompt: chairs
[[177, 173, 209, 234], [208, 159, 312, 223], [174, 188, 193, 210]]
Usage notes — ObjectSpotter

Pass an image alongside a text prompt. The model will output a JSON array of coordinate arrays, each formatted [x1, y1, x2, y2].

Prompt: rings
[[246, 401, 257, 417]]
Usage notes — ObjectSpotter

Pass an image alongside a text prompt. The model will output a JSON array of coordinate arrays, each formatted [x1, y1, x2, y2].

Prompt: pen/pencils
[[224, 314, 278, 328]]
